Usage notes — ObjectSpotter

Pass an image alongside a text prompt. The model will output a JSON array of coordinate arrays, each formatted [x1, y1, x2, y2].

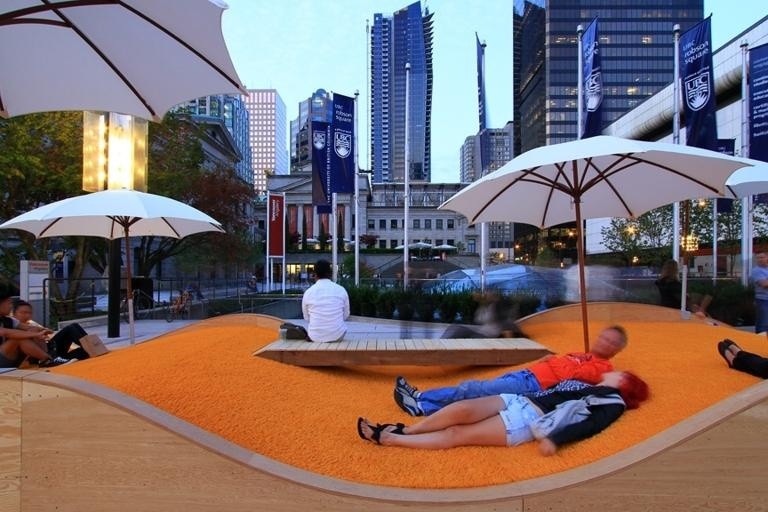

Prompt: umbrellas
[[306, 237, 360, 245], [0, 1, 254, 127], [436, 134, 757, 353], [1, 186, 225, 344], [394, 239, 456, 258], [680, 153, 768, 270]]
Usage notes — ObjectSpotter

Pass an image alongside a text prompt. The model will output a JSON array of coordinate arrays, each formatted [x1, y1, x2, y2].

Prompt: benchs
[[251, 336, 558, 367]]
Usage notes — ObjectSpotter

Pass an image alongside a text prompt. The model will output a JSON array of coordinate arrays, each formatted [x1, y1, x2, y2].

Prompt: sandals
[[357, 416, 382, 446], [377, 423, 407, 435]]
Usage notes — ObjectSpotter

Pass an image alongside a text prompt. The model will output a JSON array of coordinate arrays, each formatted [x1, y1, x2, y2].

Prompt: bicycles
[[161, 288, 193, 324], [122, 286, 154, 326]]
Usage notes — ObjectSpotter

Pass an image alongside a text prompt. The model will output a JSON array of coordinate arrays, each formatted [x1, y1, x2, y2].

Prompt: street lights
[[514, 239, 522, 265]]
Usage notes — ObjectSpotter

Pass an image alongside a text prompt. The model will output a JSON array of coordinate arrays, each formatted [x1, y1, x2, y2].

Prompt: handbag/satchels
[[529, 392, 627, 444]]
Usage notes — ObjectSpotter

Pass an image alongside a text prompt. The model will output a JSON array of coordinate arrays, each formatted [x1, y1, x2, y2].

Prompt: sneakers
[[394, 386, 420, 418], [37, 355, 79, 370], [396, 375, 422, 401]]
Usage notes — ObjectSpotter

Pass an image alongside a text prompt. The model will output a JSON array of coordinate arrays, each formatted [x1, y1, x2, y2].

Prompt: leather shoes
[[724, 338, 744, 358], [717, 341, 736, 368]]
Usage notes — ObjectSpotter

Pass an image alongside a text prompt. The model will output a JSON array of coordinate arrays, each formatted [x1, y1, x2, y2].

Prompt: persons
[[1, 290, 61, 367], [653, 259, 687, 309], [717, 338, 768, 379], [12, 299, 93, 360], [751, 252, 768, 333], [356, 369, 648, 455], [246, 276, 257, 293], [394, 326, 629, 417], [302, 259, 351, 344]]
